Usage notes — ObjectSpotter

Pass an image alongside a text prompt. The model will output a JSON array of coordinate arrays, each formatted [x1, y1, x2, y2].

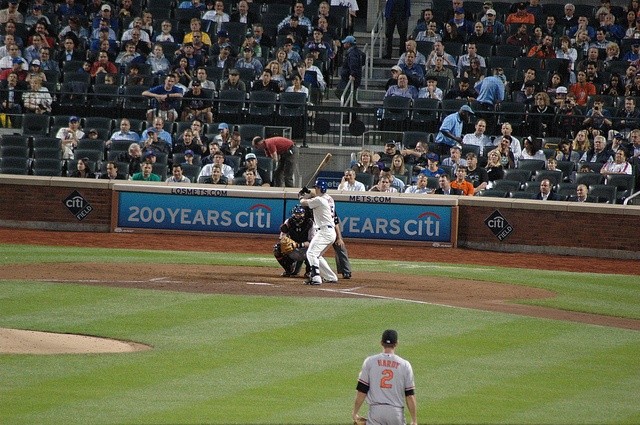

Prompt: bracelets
[[167, 93, 169, 98]]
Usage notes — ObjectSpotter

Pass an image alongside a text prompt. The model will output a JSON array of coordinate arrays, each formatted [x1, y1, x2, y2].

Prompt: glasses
[[398, 77, 407, 80], [466, 152, 477, 158], [9, 48, 18, 51], [483, 3, 492, 6], [192, 36, 201, 38], [537, 97, 543, 100], [41, 52, 49, 55], [600, 13, 607, 16], [162, 24, 172, 27], [192, 84, 200, 86]]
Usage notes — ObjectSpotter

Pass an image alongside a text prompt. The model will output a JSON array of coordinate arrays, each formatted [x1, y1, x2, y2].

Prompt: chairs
[[1, 1, 349, 185], [354, 2, 640, 206]]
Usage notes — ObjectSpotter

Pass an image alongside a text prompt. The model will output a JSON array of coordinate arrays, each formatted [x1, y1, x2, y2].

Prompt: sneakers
[[304, 271, 312, 278], [290, 270, 300, 277], [302, 279, 322, 285], [281, 268, 294, 276], [343, 271, 351, 279], [342, 116, 355, 125], [322, 277, 338, 283], [382, 54, 391, 59]]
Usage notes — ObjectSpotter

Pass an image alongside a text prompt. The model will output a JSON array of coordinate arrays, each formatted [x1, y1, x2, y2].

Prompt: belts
[[477, 101, 490, 105], [318, 225, 333, 230]]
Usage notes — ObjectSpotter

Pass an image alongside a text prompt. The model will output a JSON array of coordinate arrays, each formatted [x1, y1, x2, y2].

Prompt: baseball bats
[[305, 153, 332, 188]]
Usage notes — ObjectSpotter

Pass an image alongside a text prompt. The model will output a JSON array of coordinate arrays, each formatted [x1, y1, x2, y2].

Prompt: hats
[[244, 46, 254, 53], [486, 9, 496, 16], [69, 116, 79, 123], [290, 13, 299, 21], [454, 6, 465, 14], [217, 122, 229, 130], [13, 58, 24, 63], [460, 105, 474, 114], [385, 139, 396, 146], [9, 0, 19, 4], [517, 2, 526, 10], [192, 78, 201, 84], [185, 150, 195, 156], [314, 26, 323, 33], [229, 69, 239, 75], [342, 35, 357, 44], [245, 153, 257, 160], [311, 45, 319, 52], [427, 153, 438, 161], [32, 4, 42, 10], [426, 76, 438, 81], [382, 330, 398, 344], [613, 133, 624, 140], [348, 120, 365, 137], [296, 61, 306, 68], [31, 60, 40, 66], [245, 33, 253, 39], [390, 64, 403, 72], [147, 127, 157, 133], [556, 86, 568, 94], [101, 4, 111, 12], [314, 117, 330, 135], [631, 39, 640, 48], [145, 151, 155, 157], [218, 30, 230, 38], [89, 129, 98, 133], [99, 26, 109, 31], [220, 41, 232, 49]]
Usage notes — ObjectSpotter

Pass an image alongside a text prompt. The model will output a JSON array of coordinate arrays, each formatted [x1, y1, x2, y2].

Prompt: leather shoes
[[283, 142, 296, 155]]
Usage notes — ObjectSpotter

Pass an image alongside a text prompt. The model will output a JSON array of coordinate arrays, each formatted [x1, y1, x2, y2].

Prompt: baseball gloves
[[280, 237, 298, 257]]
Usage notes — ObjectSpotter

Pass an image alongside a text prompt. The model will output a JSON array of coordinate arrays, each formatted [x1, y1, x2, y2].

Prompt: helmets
[[311, 179, 328, 193], [292, 204, 306, 224]]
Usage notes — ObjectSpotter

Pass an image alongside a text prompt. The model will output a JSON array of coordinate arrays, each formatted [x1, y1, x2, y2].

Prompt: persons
[[55, 116, 297, 188], [299, 180, 339, 286], [0, 0, 358, 117], [337, 117, 640, 206], [297, 187, 352, 280], [182, 79, 212, 123], [352, 329, 417, 425], [141, 74, 183, 121], [381, 0, 640, 118], [273, 206, 316, 278], [335, 36, 364, 123]]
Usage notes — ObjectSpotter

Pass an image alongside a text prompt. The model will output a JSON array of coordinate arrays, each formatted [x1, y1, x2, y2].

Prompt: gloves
[[298, 191, 305, 200], [302, 187, 311, 194]]
